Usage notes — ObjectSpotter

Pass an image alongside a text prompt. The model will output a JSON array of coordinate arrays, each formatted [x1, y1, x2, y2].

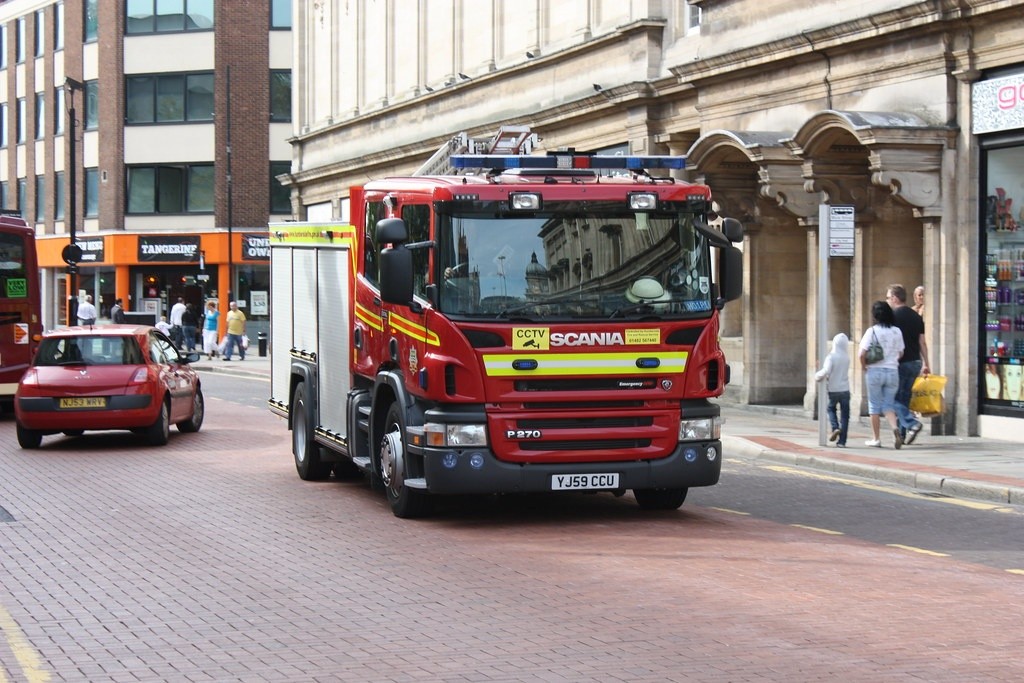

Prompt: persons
[[181, 302, 198, 353], [885, 283, 931, 445], [422, 222, 498, 284], [909, 285, 925, 318], [202, 301, 220, 360], [155, 316, 173, 348], [859, 301, 902, 450], [222, 302, 246, 361], [170, 297, 187, 350], [815, 333, 853, 448], [76, 295, 96, 326], [111, 298, 125, 324]]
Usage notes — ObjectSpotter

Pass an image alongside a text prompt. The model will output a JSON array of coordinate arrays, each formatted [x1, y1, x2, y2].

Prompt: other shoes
[[222, 358, 230, 361], [239, 356, 245, 360], [893, 427, 903, 449], [829, 429, 841, 441], [836, 442, 845, 448], [865, 439, 882, 446], [904, 421, 923, 445]]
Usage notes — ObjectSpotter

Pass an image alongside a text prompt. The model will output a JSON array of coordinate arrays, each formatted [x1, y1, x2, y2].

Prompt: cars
[[13, 326, 205, 447]]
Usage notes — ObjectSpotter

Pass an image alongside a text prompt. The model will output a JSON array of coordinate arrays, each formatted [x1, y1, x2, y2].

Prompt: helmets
[[625, 278, 669, 308]]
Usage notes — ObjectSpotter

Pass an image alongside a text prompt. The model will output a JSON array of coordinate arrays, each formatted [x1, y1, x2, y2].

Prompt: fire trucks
[[267, 125, 743, 521]]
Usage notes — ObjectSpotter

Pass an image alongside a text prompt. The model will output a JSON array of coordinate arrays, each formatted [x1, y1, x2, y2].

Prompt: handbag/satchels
[[922, 393, 947, 417], [219, 336, 228, 354], [860, 326, 884, 364], [240, 335, 251, 350], [909, 373, 948, 414]]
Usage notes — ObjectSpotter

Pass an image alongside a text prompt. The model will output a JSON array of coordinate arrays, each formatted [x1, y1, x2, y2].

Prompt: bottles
[[983, 187, 1024, 365]]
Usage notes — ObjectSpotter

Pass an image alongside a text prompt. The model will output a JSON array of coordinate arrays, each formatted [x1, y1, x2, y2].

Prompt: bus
[[0, 208, 43, 403]]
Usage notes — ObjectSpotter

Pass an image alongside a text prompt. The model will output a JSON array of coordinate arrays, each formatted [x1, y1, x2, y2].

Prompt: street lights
[[62, 77, 85, 344]]
